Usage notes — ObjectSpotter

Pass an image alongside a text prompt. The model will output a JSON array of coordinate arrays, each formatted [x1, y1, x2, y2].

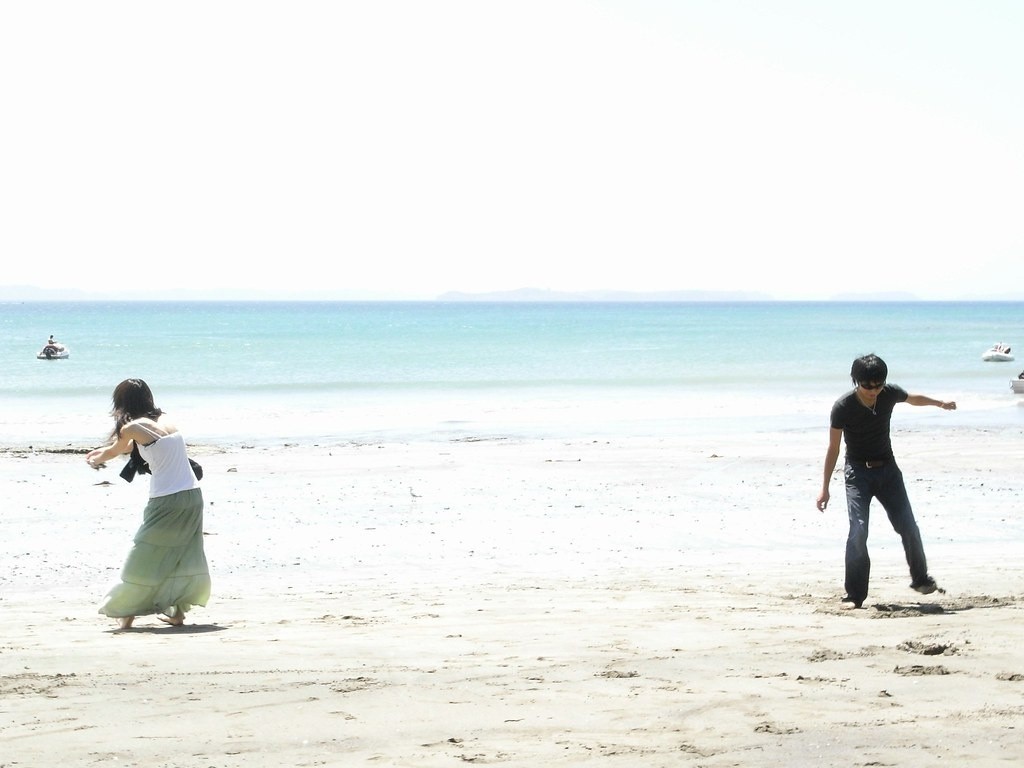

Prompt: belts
[[846, 459, 895, 468]]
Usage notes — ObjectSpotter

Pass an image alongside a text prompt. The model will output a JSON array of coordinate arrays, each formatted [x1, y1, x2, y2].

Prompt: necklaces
[[856, 389, 877, 415]]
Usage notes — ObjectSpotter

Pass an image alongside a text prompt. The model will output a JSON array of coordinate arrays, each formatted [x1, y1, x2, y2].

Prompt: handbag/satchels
[[120, 438, 203, 482]]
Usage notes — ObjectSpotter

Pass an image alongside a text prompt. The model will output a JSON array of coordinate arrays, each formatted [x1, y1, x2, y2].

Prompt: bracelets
[[90, 459, 96, 467], [939, 401, 944, 407]]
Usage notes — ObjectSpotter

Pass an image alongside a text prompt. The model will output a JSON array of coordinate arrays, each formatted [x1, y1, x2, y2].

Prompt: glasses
[[861, 381, 885, 390]]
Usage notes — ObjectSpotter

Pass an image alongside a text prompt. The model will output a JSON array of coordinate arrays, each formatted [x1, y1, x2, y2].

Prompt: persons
[[86, 379, 212, 628], [48, 334, 57, 344], [994, 341, 1003, 351], [816, 355, 956, 611]]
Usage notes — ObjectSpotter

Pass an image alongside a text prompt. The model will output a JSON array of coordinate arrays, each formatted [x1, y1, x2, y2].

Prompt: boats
[[981, 343, 1015, 362], [36, 342, 70, 361], [1008, 378, 1024, 394]]
